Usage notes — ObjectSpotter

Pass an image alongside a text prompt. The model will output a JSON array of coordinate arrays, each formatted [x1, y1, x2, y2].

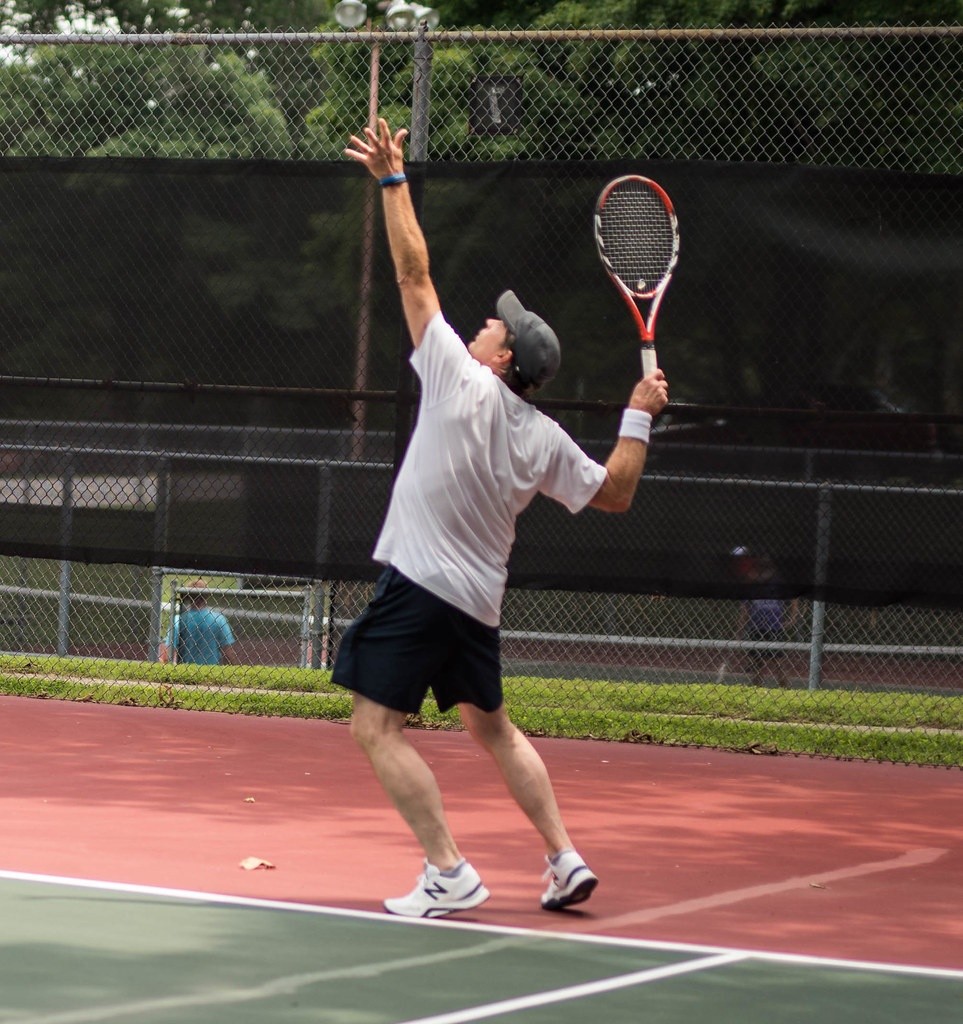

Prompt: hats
[[188, 580, 208, 588], [497, 290, 561, 386]]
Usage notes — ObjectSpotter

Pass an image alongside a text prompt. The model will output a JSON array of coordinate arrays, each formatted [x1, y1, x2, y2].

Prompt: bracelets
[[617, 406, 654, 446], [377, 172, 408, 186]]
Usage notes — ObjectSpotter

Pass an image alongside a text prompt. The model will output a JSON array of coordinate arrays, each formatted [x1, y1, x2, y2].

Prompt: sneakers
[[384, 858, 490, 917], [540, 849, 599, 909]]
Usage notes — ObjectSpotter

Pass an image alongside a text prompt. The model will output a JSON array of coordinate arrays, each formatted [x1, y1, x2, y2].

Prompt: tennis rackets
[[594, 175, 682, 379]]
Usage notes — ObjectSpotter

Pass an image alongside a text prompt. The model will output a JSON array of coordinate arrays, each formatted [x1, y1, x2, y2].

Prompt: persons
[[339, 116, 670, 919], [160, 580, 243, 665], [730, 546, 802, 691]]
